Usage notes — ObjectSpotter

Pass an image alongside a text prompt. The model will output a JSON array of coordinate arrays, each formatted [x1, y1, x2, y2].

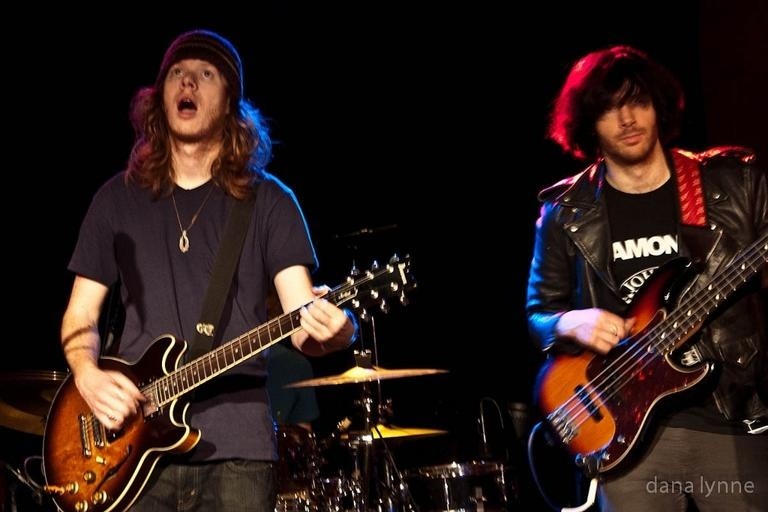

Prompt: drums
[[272, 420, 323, 512], [377, 471, 421, 512], [307, 473, 366, 512], [420, 460, 509, 512]]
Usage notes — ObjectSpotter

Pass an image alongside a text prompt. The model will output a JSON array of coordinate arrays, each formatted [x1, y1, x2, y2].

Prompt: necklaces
[[164, 175, 216, 254]]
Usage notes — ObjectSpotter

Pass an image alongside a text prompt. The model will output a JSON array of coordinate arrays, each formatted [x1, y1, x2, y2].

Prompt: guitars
[[532, 220, 768, 476], [42, 249, 416, 512]]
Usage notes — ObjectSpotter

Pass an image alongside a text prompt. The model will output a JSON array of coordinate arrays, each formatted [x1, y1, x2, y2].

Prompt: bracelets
[[342, 308, 361, 346]]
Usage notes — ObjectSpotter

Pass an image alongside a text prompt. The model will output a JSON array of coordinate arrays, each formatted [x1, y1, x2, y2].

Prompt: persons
[[59, 29, 358, 512], [523, 44, 768, 512]]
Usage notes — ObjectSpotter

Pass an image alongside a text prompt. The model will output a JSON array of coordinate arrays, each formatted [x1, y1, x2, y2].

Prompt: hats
[[157, 30, 243, 110]]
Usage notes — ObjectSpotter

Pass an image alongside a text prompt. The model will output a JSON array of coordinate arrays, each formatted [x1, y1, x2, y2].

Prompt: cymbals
[[281, 360, 448, 386], [341, 423, 448, 443]]
